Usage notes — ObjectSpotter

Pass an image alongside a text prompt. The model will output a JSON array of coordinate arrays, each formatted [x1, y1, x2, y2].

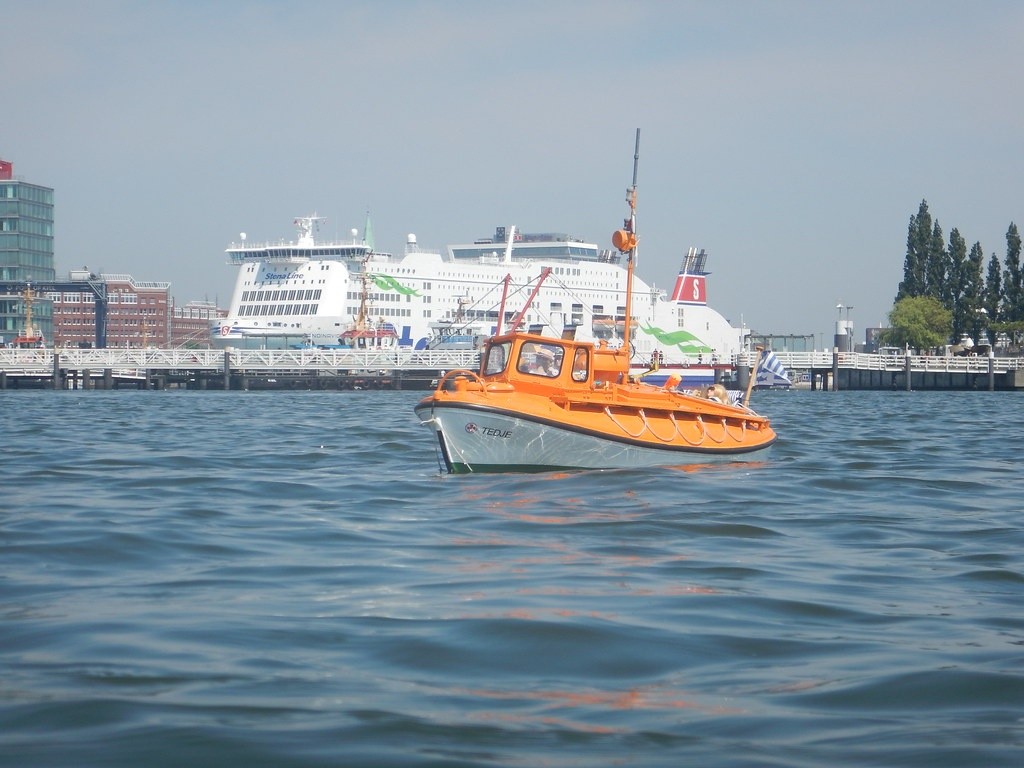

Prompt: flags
[[753, 350, 792, 386]]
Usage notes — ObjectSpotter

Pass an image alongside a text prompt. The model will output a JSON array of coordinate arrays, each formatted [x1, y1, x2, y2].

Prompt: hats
[[534, 349, 554, 361], [554, 351, 562, 357]]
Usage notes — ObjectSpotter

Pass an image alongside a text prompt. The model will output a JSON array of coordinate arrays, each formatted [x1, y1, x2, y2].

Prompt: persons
[[707, 385, 727, 404], [534, 349, 559, 376]]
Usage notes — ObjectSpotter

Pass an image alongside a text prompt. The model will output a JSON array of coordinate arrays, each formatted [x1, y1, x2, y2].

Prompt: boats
[[290, 259, 411, 349], [426, 298, 491, 350], [414, 127, 779, 474]]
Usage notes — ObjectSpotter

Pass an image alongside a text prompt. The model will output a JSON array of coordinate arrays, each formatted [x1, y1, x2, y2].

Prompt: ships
[[209, 211, 751, 390]]
